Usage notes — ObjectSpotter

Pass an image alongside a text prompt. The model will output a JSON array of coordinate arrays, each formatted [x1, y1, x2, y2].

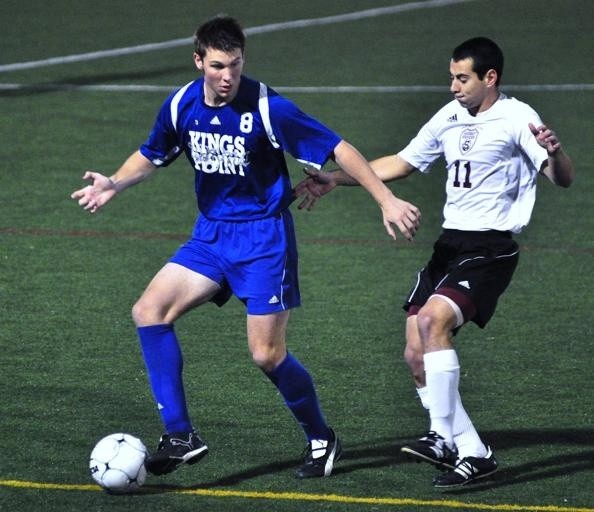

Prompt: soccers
[[89, 434, 149, 494]]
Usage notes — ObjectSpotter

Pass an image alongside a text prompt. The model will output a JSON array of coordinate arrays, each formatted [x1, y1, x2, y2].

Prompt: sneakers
[[296, 425, 346, 479], [431, 445, 500, 488], [399, 430, 460, 472], [144, 429, 211, 473]]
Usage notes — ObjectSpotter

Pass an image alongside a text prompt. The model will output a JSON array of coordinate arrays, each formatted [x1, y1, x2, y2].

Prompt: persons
[[68, 17, 423, 477], [289, 37, 572, 491]]
